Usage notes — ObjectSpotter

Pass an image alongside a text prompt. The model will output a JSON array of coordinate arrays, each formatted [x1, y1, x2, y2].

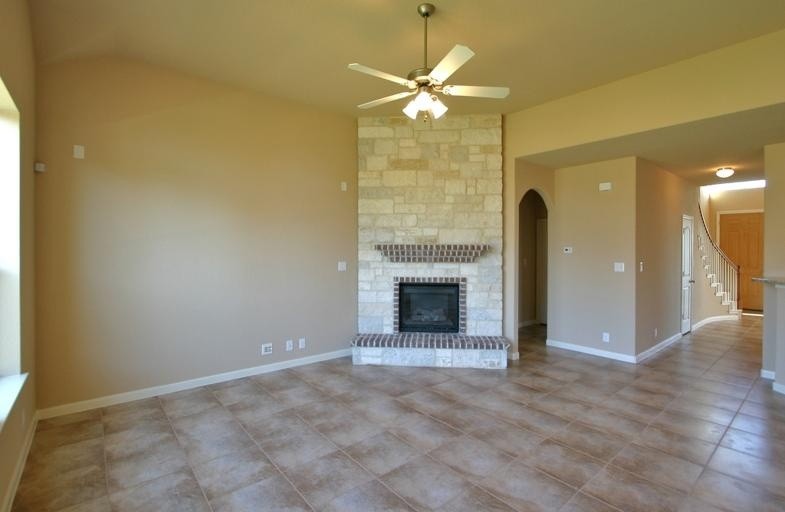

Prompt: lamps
[[399, 84, 448, 121], [715, 167, 736, 180]]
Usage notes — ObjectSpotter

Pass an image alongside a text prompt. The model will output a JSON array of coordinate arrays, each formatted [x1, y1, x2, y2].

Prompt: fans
[[344, 4, 512, 121]]
